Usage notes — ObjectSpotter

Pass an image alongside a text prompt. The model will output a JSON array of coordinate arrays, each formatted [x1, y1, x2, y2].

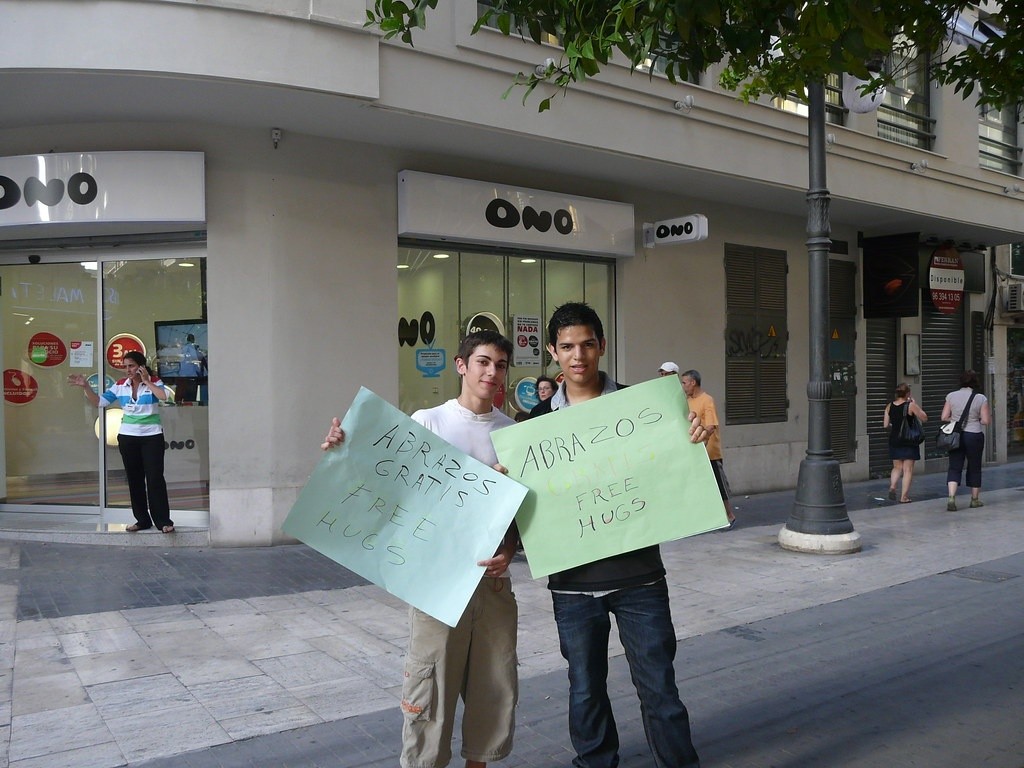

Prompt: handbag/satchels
[[896, 401, 926, 447], [935, 420, 961, 452]]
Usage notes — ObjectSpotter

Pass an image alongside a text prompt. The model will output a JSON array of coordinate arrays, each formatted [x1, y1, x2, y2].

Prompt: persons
[[320, 331, 523, 768], [941, 369, 991, 511], [883, 382, 928, 503], [513, 373, 557, 559], [656, 360, 738, 529], [490, 299, 712, 768], [66, 351, 176, 534], [1007, 377, 1022, 428]]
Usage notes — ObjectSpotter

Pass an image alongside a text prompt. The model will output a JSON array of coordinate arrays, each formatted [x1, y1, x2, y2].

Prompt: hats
[[657, 361, 680, 374]]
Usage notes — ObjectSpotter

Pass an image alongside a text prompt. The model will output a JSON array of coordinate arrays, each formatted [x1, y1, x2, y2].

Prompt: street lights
[[778, 18, 886, 556]]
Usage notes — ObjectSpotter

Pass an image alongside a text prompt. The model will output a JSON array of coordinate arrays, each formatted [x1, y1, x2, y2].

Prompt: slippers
[[889, 490, 896, 501], [162, 523, 175, 534], [728, 518, 737, 529], [126, 522, 151, 532], [899, 497, 912, 503]]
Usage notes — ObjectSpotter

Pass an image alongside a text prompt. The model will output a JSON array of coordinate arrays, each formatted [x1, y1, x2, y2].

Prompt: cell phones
[[137, 365, 145, 376]]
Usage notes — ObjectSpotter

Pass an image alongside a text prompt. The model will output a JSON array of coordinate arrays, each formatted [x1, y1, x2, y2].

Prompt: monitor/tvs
[[154, 319, 208, 384]]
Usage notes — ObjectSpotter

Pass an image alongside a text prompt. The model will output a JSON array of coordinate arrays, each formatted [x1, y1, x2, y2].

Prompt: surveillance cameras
[[28, 251, 41, 263]]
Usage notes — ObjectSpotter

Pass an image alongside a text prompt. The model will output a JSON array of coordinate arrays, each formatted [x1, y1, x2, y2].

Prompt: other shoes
[[970, 496, 984, 507], [947, 496, 957, 511]]
